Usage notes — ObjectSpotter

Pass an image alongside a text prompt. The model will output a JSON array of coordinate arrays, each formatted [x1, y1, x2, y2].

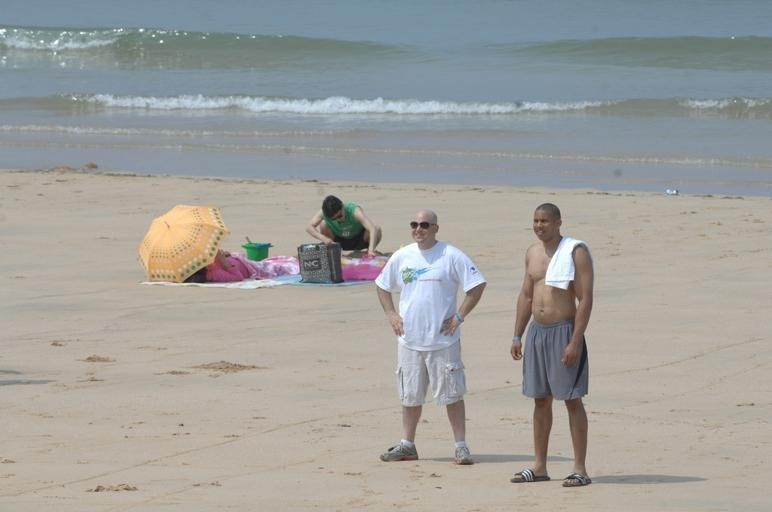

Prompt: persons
[[508, 202, 596, 486], [307, 194, 383, 260], [185, 249, 300, 283], [373, 209, 488, 464]]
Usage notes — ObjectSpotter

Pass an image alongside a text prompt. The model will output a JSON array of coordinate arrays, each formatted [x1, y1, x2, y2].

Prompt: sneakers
[[380, 442, 418, 461], [455, 446, 473, 465]]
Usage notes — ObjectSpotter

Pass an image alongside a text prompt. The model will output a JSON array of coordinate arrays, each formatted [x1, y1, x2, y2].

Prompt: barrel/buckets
[[241, 241, 272, 260]]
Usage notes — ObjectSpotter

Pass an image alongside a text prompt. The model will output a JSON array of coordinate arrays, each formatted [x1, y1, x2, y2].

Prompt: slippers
[[511, 468, 550, 482], [563, 472, 592, 487]]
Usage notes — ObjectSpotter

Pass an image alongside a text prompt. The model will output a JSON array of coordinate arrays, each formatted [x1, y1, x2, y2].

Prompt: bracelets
[[452, 311, 466, 325], [511, 336, 521, 343]]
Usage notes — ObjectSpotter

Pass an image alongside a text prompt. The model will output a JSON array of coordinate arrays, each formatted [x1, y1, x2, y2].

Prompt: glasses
[[331, 210, 343, 221], [410, 222, 436, 229]]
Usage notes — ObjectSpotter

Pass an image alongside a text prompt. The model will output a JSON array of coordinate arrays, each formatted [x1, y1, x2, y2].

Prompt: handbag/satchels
[[297, 242, 344, 284]]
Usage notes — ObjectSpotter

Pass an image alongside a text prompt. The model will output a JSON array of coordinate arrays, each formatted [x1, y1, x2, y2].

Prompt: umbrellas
[[138, 205, 230, 283]]
